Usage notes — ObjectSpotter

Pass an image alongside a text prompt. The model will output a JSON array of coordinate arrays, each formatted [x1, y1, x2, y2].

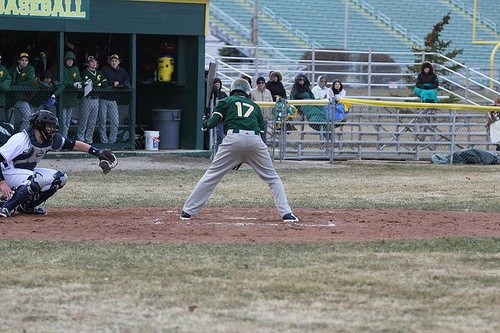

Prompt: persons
[[0, 55, 12, 121], [242, 75, 273, 144], [57, 51, 90, 139], [98, 54, 130, 143], [291, 74, 315, 115], [329, 79, 347, 126], [312, 75, 331, 99], [487, 98, 500, 151], [7, 53, 35, 131], [180, 78, 298, 221], [77, 56, 109, 144], [415, 61, 439, 114], [266, 70, 296, 130], [33, 71, 65, 114], [0, 110, 118, 218], [214, 78, 228, 143]]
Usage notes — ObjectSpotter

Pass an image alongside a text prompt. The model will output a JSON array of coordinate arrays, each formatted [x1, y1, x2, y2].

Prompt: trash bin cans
[[153, 109, 182, 150]]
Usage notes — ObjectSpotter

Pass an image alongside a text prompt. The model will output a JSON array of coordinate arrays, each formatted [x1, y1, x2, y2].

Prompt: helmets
[[230, 78, 253, 97], [29, 110, 60, 141]]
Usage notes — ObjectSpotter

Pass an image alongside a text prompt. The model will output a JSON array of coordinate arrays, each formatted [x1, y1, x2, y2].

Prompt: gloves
[[200, 113, 210, 125], [73, 81, 83, 89]]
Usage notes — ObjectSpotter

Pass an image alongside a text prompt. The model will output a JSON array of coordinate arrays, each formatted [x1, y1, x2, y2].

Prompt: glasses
[[258, 82, 264, 85], [299, 78, 306, 81]]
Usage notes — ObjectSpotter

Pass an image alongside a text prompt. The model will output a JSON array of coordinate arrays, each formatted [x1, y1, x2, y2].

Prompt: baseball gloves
[[96, 147, 119, 175]]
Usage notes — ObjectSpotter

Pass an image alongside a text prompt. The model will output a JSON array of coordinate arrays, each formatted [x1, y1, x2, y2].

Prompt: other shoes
[[0, 206, 11, 218], [17, 205, 46, 216], [283, 213, 300, 223], [180, 211, 191, 220]]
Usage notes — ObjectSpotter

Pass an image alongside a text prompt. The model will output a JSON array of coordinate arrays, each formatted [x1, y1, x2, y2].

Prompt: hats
[[257, 77, 265, 83], [109, 54, 119, 61], [18, 52, 30, 60], [87, 55, 97, 62], [214, 78, 221, 84]]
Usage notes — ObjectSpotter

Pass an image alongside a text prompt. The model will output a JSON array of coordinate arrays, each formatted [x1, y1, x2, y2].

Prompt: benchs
[[70, 100, 142, 133], [255, 97, 500, 149]]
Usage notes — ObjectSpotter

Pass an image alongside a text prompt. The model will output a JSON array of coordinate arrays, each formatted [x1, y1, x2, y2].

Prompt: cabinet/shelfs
[[138, 34, 188, 87]]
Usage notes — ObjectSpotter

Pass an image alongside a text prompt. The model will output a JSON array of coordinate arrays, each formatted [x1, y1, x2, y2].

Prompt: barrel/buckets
[[151, 108, 183, 150], [158, 56, 175, 82], [144, 130, 160, 150]]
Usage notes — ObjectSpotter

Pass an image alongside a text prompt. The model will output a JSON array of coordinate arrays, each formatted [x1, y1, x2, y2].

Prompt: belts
[[225, 129, 261, 137]]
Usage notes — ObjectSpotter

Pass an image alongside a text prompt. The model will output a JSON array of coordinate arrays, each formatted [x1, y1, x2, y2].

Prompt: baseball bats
[[200, 60, 216, 131]]
